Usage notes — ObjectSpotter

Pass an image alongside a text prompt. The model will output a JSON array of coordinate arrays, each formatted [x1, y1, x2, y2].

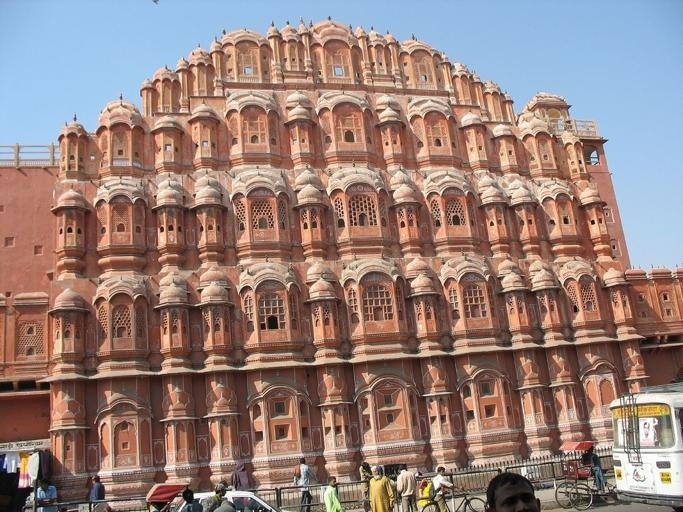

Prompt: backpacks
[[419, 479, 440, 500]]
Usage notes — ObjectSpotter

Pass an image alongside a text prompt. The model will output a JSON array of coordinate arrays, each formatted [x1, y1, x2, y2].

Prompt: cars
[[159, 490, 298, 512]]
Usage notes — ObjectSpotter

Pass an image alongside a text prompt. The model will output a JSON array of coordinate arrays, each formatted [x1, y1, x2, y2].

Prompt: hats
[[214, 484, 232, 492]]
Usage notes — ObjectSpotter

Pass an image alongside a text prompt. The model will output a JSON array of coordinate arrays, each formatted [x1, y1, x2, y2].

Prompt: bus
[[609, 382, 683, 511]]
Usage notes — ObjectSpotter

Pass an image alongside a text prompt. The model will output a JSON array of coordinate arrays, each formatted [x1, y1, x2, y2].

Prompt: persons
[[358, 461, 374, 512], [293, 458, 317, 512], [396, 464, 419, 512], [36, 478, 58, 512], [641, 421, 652, 440], [323, 475, 345, 512], [429, 467, 464, 512], [367, 465, 395, 512], [202, 482, 226, 511], [484, 472, 541, 512], [180, 488, 202, 511], [581, 445, 609, 495], [88, 475, 105, 512]]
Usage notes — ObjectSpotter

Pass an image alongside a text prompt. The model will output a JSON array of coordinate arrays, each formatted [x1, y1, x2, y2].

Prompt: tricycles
[[555, 442, 619, 510]]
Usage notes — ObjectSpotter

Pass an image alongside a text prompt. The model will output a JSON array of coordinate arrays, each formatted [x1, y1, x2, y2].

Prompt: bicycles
[[422, 486, 487, 512]]
